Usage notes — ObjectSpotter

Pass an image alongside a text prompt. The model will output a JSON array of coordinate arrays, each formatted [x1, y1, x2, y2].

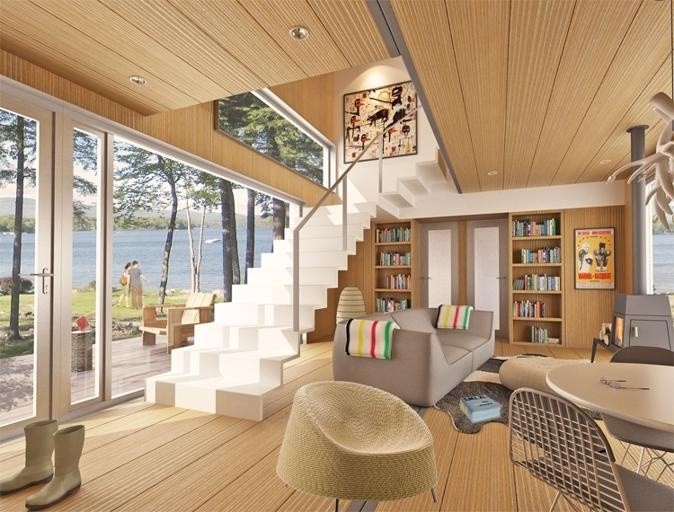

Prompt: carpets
[[431, 355, 603, 437]]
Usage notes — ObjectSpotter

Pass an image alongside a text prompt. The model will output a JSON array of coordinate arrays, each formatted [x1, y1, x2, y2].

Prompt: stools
[[501, 357, 591, 394]]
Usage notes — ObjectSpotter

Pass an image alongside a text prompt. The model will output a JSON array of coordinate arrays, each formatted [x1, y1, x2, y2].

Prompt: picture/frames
[[574, 228, 616, 290], [342, 79, 418, 164]]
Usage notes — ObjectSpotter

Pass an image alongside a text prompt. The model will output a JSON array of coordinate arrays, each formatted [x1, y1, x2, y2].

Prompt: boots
[[25, 426, 85, 511], [0, 421, 58, 495]]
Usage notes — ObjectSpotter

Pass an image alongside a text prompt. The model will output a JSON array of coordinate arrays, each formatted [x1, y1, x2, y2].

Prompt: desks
[[545, 362, 674, 432]]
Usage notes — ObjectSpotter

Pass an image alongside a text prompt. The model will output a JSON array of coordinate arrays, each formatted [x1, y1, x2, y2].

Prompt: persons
[[578, 243, 593, 272], [128, 260, 148, 309], [117, 261, 132, 307], [593, 243, 611, 272]]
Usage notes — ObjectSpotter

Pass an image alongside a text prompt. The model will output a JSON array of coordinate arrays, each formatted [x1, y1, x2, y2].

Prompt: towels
[[344, 319, 400, 360], [435, 305, 473, 330]]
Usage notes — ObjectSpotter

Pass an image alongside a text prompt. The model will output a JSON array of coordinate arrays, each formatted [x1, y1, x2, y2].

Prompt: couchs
[[332, 307, 496, 407]]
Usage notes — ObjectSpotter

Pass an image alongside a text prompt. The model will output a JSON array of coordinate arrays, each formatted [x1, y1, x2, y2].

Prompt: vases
[[335, 286, 366, 325]]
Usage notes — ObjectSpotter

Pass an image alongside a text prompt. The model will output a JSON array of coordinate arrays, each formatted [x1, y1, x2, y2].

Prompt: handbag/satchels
[[120, 276, 127, 286]]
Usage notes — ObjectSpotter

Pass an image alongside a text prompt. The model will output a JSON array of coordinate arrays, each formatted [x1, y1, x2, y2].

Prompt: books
[[459, 393, 502, 418], [513, 217, 560, 318], [531, 326, 560, 344], [376, 226, 412, 313], [458, 401, 501, 423]]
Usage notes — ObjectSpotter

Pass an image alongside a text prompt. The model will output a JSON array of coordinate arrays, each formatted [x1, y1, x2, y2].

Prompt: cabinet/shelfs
[[373, 220, 420, 312], [507, 213, 565, 347]]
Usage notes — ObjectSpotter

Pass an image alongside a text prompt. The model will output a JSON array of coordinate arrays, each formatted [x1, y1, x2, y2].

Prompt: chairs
[[507, 387, 674, 512], [140, 292, 216, 353], [602, 346, 674, 474], [277, 380, 437, 512]]
